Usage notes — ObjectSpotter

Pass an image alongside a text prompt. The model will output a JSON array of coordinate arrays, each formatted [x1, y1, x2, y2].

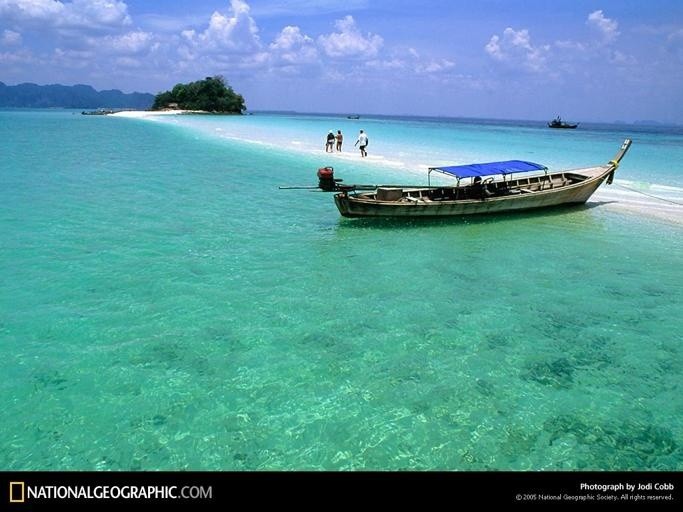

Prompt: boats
[[546, 114, 581, 131], [328, 138, 632, 219]]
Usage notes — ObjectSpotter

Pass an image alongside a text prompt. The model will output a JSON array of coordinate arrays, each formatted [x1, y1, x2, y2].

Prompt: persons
[[354, 129, 368, 157], [326, 130, 335, 152], [335, 130, 343, 152]]
